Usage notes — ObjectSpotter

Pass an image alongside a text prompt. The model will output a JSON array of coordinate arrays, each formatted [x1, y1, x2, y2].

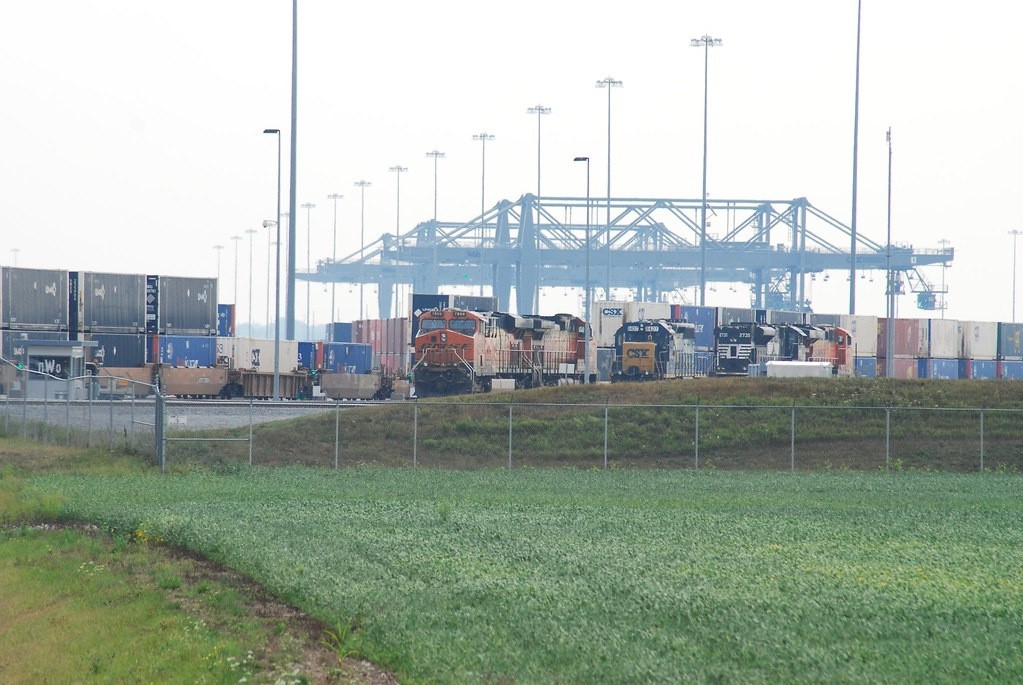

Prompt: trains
[[712, 322, 855, 379], [410, 306, 593, 394], [608, 317, 694, 383], [1, 361, 412, 400]]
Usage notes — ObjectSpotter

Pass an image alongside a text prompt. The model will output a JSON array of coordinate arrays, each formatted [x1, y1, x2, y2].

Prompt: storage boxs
[[594, 300, 1023, 380], [0, 266, 408, 379]]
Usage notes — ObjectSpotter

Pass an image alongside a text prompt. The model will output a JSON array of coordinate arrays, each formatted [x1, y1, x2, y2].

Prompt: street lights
[[302, 202, 316, 342], [473, 133, 495, 296], [575, 156, 590, 388], [426, 149, 445, 295], [390, 164, 409, 317], [326, 194, 345, 345], [352, 180, 373, 321], [885, 127, 892, 390], [530, 105, 551, 316], [1007, 229, 1019, 322], [215, 214, 288, 335], [688, 35, 721, 303], [263, 129, 284, 399], [596, 77, 624, 301]]
